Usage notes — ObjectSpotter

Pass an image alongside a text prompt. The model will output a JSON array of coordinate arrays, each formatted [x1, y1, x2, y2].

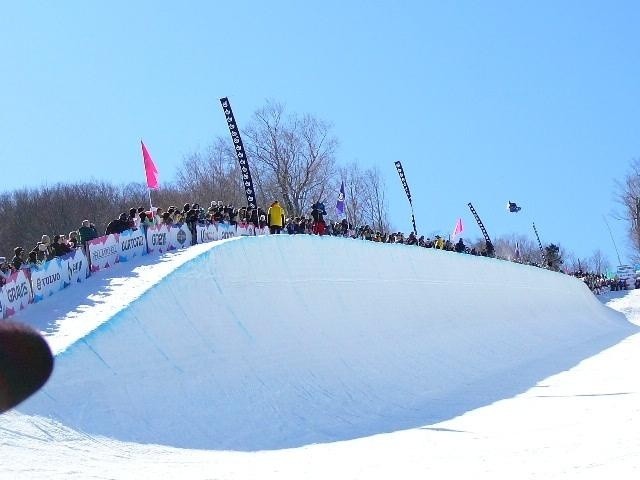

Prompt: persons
[[268, 201, 496, 258], [497, 254, 640, 296], [104, 201, 267, 246], [0, 220, 98, 296]]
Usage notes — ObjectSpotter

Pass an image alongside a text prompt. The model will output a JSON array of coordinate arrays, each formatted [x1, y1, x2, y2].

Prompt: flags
[[141, 139, 160, 193], [334, 179, 346, 216], [514, 242, 521, 258], [504, 200, 522, 216]]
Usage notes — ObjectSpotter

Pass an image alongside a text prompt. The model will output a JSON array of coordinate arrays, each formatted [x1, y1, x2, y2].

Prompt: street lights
[[349, 181, 355, 198]]
